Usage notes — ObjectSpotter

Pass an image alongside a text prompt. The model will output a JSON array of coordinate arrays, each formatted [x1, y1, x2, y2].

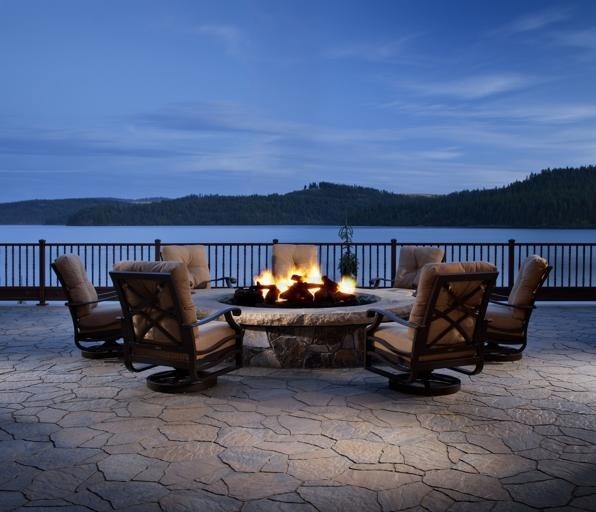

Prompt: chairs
[[483, 255, 554, 362], [109, 261, 246, 394], [272, 244, 319, 277], [50, 252, 125, 358], [159, 244, 237, 303], [363, 260, 499, 397], [370, 245, 445, 296]]
